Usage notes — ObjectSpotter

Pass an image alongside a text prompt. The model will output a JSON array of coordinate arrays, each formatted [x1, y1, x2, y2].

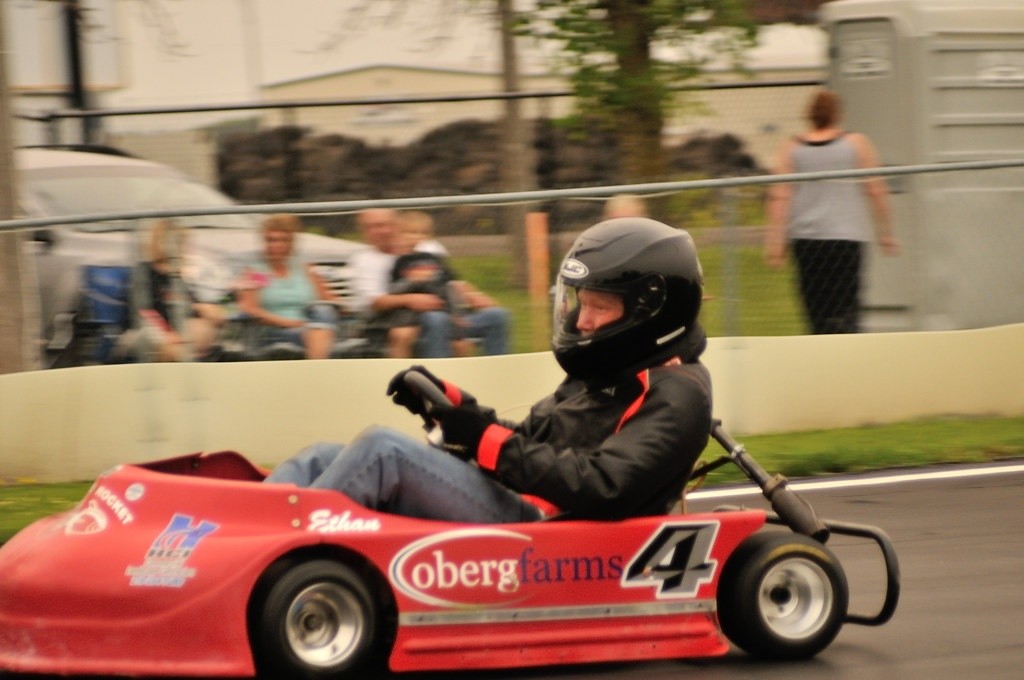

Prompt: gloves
[[429, 407, 491, 446], [386, 365, 446, 404]]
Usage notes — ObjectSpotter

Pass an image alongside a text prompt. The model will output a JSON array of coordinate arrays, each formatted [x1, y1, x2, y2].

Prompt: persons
[[110, 219, 223, 363], [766, 92, 900, 335], [351, 209, 510, 359], [230, 214, 339, 360], [264, 216, 712, 523]]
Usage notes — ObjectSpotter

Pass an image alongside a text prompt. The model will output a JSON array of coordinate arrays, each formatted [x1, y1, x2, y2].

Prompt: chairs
[[47, 266, 133, 371]]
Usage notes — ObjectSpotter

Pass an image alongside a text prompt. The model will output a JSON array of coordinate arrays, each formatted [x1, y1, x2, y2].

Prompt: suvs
[[0, 149, 400, 375]]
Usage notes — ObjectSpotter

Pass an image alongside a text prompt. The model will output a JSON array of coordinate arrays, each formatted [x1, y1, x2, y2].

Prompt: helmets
[[551, 216, 704, 390]]
